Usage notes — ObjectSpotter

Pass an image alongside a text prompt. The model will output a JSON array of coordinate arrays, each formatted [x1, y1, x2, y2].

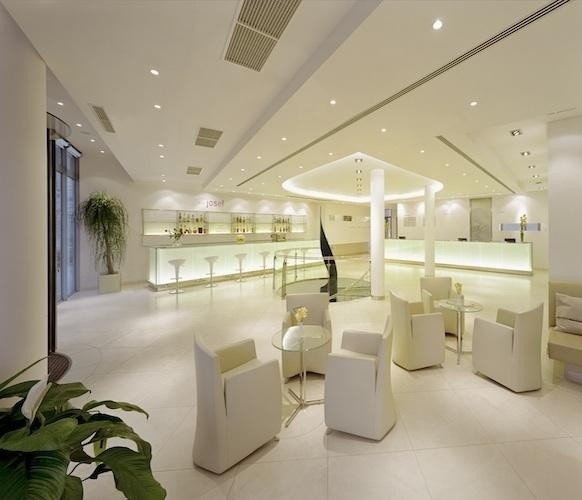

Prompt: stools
[[234, 252, 246, 282], [259, 251, 269, 278], [168, 258, 186, 294], [205, 255, 218, 287]]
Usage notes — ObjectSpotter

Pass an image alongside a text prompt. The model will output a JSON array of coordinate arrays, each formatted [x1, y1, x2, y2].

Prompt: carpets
[[48, 352, 73, 383]]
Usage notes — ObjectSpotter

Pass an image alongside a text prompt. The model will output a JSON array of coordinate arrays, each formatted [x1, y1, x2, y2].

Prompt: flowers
[[293, 304, 309, 321], [454, 281, 463, 296], [0, 354, 166, 500]]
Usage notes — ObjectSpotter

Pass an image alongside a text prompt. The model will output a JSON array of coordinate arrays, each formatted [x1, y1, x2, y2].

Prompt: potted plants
[[77, 190, 128, 294]]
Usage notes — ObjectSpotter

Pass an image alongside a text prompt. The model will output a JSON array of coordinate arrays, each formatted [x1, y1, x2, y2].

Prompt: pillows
[[556, 293, 582, 336]]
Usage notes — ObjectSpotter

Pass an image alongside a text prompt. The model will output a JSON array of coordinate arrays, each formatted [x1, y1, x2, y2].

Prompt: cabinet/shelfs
[[141, 209, 306, 246]]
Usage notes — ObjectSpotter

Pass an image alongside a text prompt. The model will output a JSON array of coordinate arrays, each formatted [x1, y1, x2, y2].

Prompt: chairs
[[420, 277, 465, 337], [325, 313, 397, 441], [281, 291, 333, 379], [191, 336, 282, 475], [472, 304, 544, 393], [388, 289, 445, 371], [547, 281, 582, 387]]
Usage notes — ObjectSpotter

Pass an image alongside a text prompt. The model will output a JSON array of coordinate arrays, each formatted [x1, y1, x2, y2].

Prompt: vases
[[458, 294, 464, 306]]
